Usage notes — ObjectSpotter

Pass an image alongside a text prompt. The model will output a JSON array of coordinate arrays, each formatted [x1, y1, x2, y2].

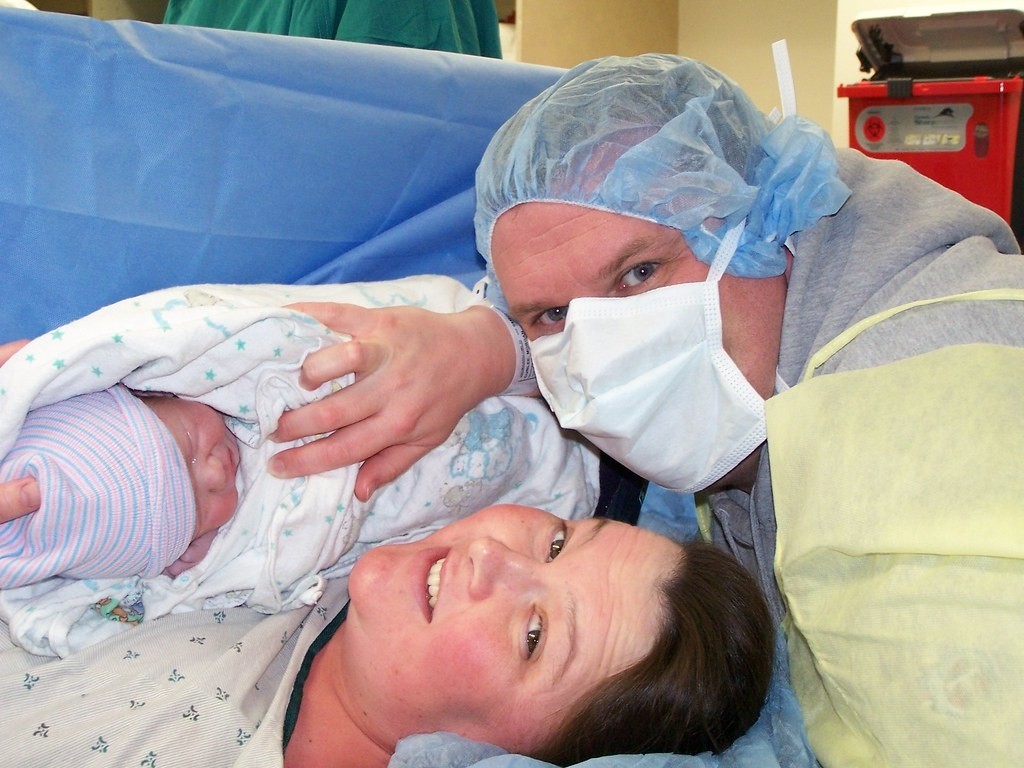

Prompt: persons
[[0, 304, 776, 768], [472, 52, 1023, 767], [0, 283, 599, 658]]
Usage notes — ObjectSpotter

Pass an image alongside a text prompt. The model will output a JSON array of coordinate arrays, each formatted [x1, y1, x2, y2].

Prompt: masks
[[527, 216, 797, 491]]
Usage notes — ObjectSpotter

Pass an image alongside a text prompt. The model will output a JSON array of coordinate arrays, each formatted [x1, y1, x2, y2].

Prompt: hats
[[0, 382, 197, 591]]
